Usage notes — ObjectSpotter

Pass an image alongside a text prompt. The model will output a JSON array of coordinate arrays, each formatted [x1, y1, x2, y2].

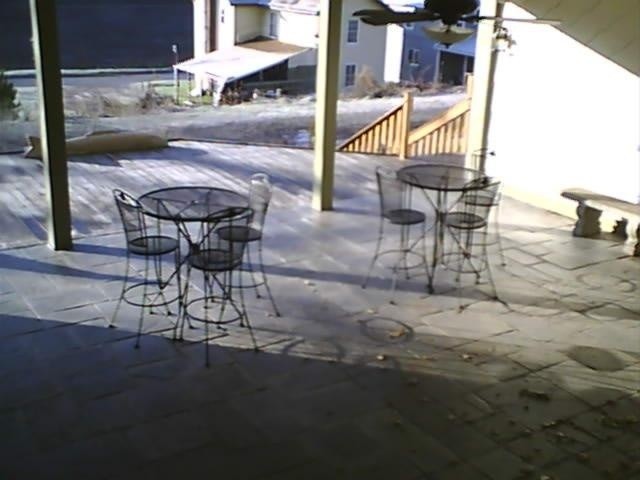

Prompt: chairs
[[361, 146, 508, 300], [108, 171, 281, 368]]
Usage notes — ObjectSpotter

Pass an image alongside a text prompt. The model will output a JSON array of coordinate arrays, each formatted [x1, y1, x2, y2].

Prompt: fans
[[352, 0, 563, 29]]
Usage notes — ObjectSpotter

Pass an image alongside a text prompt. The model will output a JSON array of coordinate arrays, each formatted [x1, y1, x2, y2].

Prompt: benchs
[[562, 187, 640, 257]]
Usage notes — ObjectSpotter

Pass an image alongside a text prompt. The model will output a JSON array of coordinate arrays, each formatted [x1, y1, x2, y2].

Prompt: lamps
[[421, 17, 475, 49]]
